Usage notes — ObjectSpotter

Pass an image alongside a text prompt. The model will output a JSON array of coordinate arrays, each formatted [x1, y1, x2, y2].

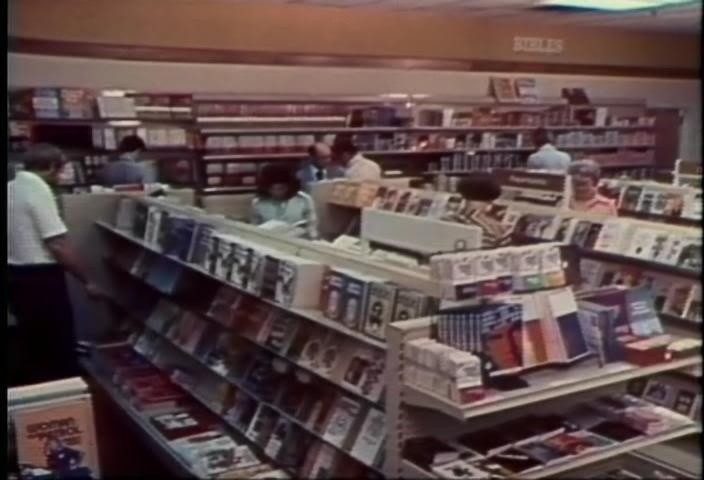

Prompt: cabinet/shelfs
[[9, 88, 200, 190], [309, 169, 703, 425], [199, 96, 661, 187], [78, 190, 435, 478], [385, 282, 703, 479]]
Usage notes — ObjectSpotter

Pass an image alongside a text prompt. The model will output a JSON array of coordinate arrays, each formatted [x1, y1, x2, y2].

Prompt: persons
[[9, 142, 110, 382]]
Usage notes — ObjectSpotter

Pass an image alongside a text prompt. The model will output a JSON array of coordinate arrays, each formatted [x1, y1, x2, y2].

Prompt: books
[[8, 377, 100, 479], [80, 173, 704, 480], [7, 85, 656, 171]]
